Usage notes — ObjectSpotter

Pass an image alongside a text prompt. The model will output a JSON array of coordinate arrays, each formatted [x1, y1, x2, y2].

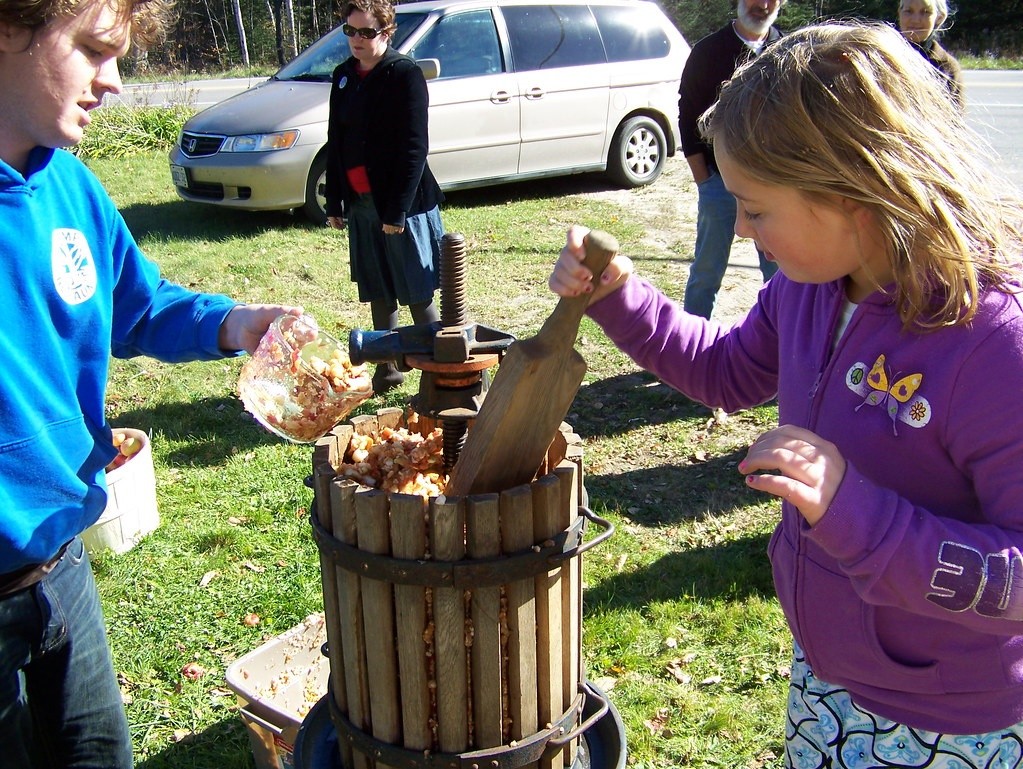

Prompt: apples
[[105, 433, 141, 472]]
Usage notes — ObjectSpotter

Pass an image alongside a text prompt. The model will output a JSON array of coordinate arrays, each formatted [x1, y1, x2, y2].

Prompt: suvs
[[168, 2, 694, 227]]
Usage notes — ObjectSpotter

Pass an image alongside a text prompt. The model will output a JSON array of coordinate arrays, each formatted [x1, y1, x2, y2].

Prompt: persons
[[678, 0, 787, 319], [0, 0, 314, 769], [326, 0, 446, 386], [549, 25, 1023, 769], [898, 0, 963, 103]]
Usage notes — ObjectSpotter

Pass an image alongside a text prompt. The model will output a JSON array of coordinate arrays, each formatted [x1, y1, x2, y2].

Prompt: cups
[[238, 314, 373, 443]]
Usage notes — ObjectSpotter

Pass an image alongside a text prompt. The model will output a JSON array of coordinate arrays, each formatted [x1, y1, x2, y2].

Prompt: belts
[[0, 536, 76, 595]]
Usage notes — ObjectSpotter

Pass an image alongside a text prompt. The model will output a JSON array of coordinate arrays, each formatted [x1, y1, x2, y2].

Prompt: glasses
[[343, 23, 384, 39]]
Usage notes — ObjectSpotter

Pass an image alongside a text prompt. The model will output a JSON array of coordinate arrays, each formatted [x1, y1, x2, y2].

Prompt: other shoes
[[372, 362, 404, 394]]
